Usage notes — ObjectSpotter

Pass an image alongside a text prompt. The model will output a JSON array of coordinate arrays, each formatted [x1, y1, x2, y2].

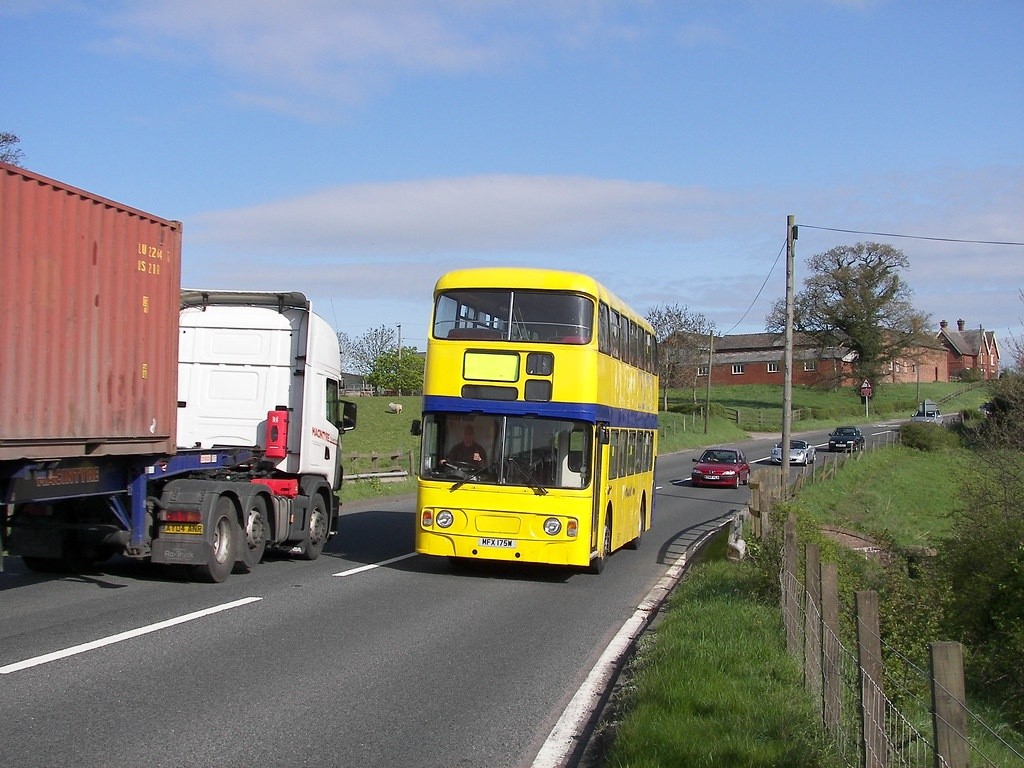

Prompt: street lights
[[911, 318, 922, 402]]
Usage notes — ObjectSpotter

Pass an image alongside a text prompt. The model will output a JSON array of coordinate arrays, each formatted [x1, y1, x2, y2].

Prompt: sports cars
[[771, 440, 816, 466]]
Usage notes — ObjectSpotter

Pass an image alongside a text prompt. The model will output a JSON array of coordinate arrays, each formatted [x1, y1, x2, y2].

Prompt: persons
[[439, 423, 488, 470], [705, 451, 719, 462]]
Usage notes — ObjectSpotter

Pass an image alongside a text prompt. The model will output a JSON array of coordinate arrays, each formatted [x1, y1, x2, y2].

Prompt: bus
[[411, 267, 658, 573]]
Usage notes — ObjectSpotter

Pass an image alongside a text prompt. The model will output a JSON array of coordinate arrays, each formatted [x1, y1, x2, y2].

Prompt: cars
[[910, 409, 943, 426], [692, 447, 750, 489], [978, 402, 992, 417], [829, 426, 865, 453]]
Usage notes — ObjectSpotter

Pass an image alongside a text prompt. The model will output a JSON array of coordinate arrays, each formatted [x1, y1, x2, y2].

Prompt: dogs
[[388, 401, 403, 413]]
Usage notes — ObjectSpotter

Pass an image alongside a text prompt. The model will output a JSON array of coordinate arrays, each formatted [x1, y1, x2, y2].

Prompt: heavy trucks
[[2, 161, 357, 582]]
